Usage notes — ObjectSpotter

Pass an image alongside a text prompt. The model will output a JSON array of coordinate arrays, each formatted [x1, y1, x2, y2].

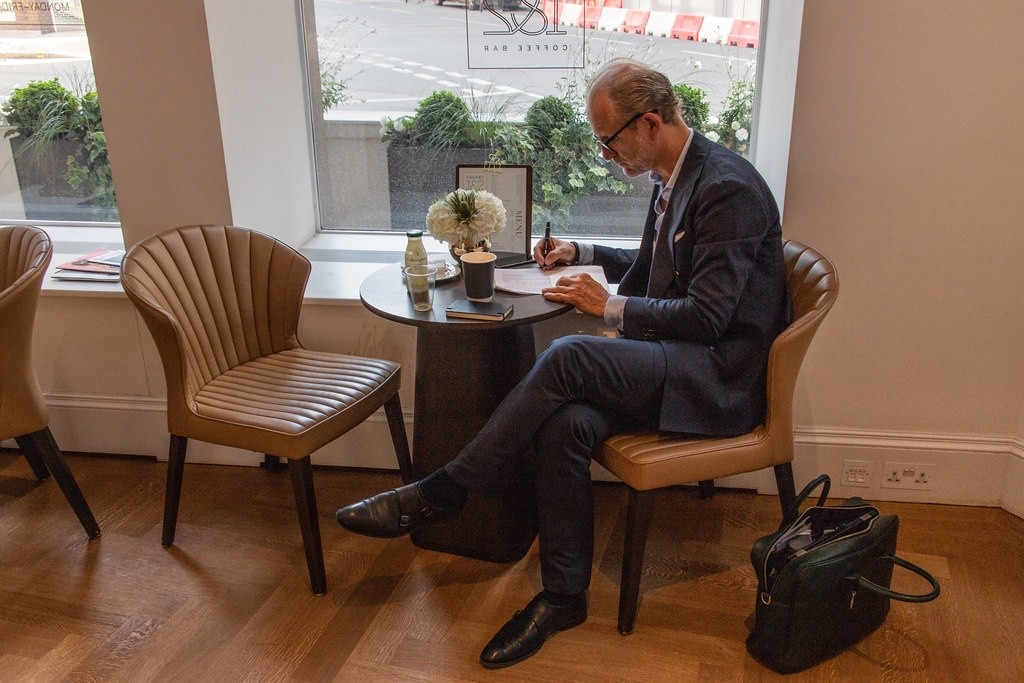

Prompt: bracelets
[[570, 241, 579, 265]]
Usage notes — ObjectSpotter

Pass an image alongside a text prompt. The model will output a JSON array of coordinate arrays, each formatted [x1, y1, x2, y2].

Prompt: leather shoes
[[480, 591, 589, 669], [336, 481, 466, 538]]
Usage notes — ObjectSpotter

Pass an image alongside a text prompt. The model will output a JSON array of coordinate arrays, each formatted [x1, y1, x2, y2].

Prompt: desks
[[361, 253, 603, 563]]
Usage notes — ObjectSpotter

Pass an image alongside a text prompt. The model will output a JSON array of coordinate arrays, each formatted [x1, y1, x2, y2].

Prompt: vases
[[447, 237, 491, 262]]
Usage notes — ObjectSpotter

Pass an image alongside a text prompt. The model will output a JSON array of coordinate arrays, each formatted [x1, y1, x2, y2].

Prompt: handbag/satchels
[[746, 475, 941, 674]]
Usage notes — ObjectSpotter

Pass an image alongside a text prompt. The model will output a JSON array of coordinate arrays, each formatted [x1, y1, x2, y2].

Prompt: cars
[[433, 0, 522, 12]]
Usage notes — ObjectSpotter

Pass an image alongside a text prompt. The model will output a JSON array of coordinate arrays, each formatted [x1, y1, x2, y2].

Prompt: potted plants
[[1, 76, 118, 198], [379, 89, 656, 211]]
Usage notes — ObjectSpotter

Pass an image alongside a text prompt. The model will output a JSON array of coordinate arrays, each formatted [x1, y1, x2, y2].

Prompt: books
[[446, 298, 513, 322]]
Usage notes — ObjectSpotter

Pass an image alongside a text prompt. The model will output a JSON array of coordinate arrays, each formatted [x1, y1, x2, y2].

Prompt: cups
[[405, 265, 438, 312], [460, 252, 497, 302]]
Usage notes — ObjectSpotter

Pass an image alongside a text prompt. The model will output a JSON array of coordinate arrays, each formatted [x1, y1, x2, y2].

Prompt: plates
[[401, 262, 461, 280]]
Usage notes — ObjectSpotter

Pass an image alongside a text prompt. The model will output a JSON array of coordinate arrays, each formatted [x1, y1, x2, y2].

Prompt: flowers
[[426, 189, 507, 247]]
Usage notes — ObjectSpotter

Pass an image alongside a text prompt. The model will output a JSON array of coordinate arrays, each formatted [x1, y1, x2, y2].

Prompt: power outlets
[[882, 462, 935, 490]]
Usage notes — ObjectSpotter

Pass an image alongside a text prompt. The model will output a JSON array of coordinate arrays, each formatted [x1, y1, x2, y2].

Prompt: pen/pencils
[[543, 221, 551, 272]]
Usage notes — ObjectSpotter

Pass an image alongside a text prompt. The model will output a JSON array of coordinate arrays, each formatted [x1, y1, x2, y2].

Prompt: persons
[[335, 57, 789, 670]]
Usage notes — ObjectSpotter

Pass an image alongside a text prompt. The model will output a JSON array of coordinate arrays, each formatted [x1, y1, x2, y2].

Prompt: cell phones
[[493, 253, 536, 268]]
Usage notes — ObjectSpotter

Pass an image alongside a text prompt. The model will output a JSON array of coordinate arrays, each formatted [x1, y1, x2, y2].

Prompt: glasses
[[592, 108, 659, 153]]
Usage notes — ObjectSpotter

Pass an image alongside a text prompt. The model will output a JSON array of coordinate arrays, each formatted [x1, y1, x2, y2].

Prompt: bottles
[[405, 230, 428, 293]]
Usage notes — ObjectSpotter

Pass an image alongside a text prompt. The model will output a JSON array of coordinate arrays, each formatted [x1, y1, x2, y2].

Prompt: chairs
[[0, 224, 102, 540], [122, 224, 412, 596], [591, 239, 839, 635]]
